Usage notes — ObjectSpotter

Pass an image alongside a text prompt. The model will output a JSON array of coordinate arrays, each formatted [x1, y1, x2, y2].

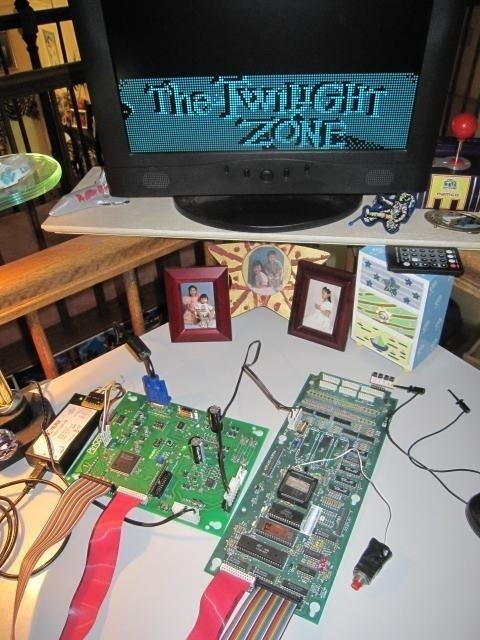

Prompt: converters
[[24, 392, 104, 474]]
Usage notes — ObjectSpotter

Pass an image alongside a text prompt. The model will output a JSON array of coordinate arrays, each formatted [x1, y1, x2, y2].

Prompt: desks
[[1, 301, 479, 640]]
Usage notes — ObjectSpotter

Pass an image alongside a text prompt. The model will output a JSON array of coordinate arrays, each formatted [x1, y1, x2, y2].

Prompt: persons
[[253, 261, 268, 289], [183, 285, 202, 325], [263, 251, 284, 284], [303, 287, 334, 333], [195, 294, 215, 322]]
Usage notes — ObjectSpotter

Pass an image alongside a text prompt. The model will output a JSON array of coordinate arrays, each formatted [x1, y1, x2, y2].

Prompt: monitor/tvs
[[67, 0, 478, 233]]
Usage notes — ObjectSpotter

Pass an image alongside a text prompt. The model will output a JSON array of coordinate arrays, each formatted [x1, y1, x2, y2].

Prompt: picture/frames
[[287, 259, 356, 352], [162, 266, 233, 343]]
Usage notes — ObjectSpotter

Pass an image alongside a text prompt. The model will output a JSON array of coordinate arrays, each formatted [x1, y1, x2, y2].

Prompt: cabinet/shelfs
[[352, 246, 454, 369]]
